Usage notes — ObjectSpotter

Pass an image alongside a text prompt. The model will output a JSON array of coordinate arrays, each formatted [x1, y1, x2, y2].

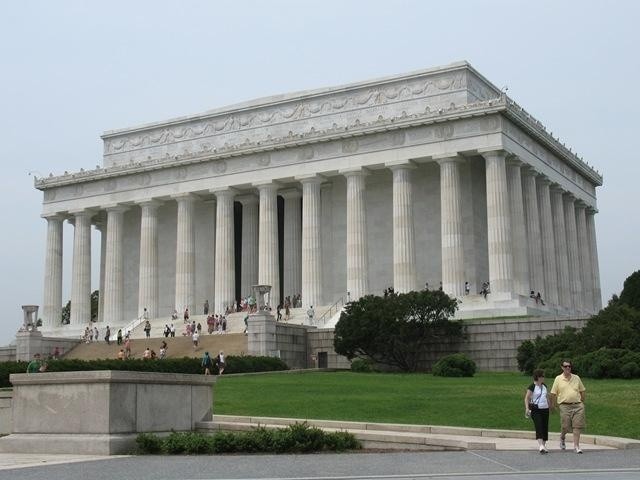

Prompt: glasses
[[563, 365, 571, 368]]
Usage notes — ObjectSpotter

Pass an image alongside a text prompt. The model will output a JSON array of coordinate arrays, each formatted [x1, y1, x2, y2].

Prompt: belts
[[561, 402, 581, 405]]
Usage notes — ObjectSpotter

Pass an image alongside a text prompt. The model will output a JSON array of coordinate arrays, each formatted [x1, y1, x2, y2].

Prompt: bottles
[[525, 410, 531, 418]]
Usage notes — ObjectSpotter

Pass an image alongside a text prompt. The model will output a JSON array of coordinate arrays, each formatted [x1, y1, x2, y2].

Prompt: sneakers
[[560, 437, 566, 449], [545, 447, 548, 453], [574, 448, 583, 454], [540, 445, 545, 453]]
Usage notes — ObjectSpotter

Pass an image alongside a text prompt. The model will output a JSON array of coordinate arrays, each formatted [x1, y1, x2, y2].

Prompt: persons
[[201, 352, 212, 376], [53, 346, 60, 360], [381, 281, 490, 299], [551, 360, 586, 453], [524, 369, 551, 453], [307, 305, 314, 324], [47, 353, 52, 361], [537, 292, 545, 305], [530, 291, 538, 304], [215, 350, 225, 375], [82, 293, 302, 360], [26, 353, 49, 373]]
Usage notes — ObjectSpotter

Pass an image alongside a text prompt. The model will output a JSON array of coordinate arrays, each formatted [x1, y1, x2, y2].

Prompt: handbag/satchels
[[529, 404, 538, 410]]
